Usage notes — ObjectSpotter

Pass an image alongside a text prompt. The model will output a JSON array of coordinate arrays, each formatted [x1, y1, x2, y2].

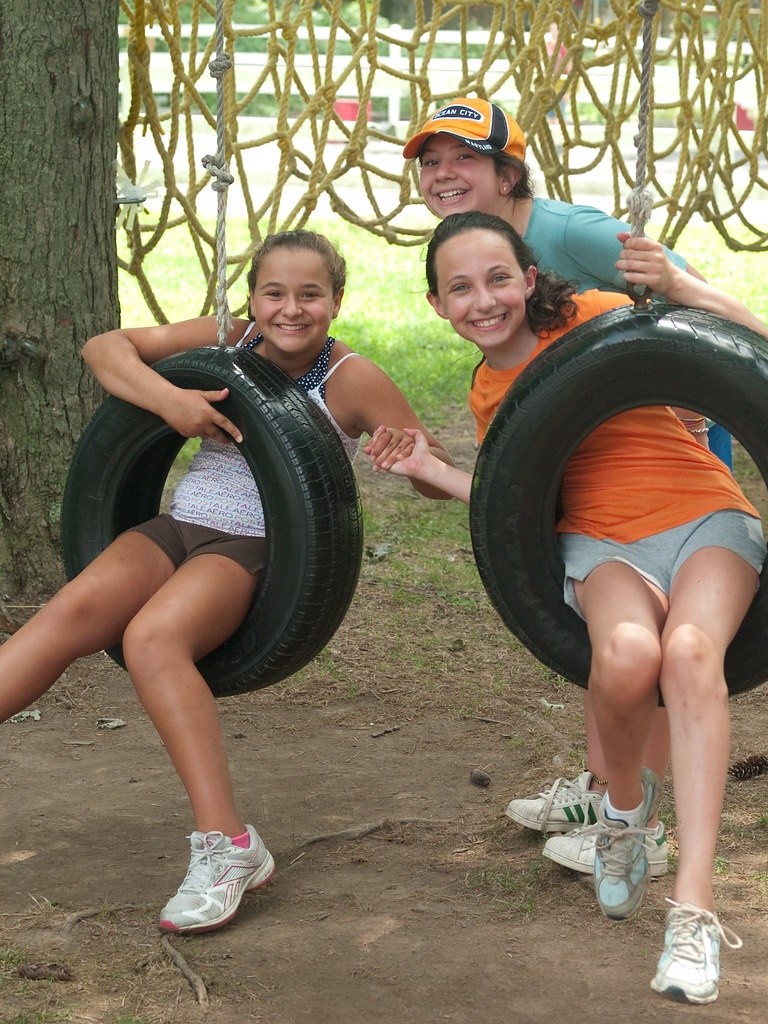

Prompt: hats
[[402, 95, 527, 171]]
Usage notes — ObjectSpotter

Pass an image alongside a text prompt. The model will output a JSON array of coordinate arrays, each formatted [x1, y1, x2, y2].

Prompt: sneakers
[[158, 823, 275, 935], [593, 768, 664, 926], [540, 820, 670, 877], [506, 773, 602, 834], [649, 903, 741, 1004]]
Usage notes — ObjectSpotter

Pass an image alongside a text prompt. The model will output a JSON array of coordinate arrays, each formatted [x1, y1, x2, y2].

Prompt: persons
[[403, 96, 732, 875], [0, 231, 454, 938], [366, 212, 768, 1006]]
